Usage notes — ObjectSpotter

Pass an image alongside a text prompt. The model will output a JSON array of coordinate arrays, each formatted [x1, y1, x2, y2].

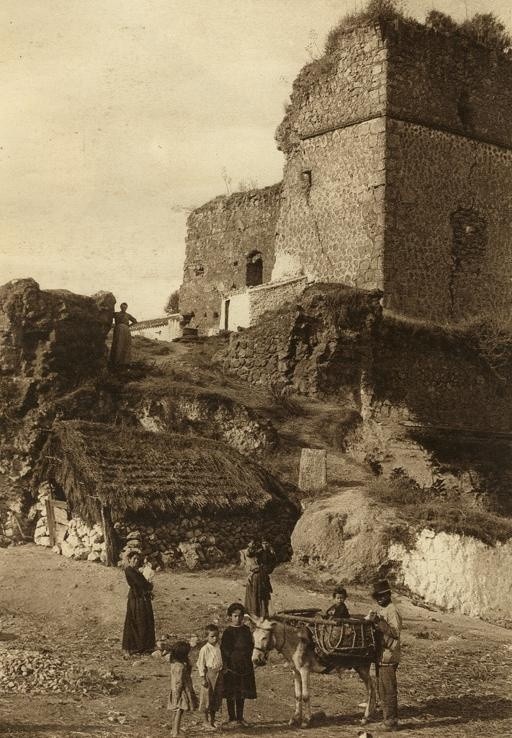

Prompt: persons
[[122, 551, 156, 650], [242, 540, 260, 582], [370, 580, 402, 730], [243, 537, 275, 617], [327, 587, 351, 621], [111, 303, 137, 365], [167, 641, 197, 737], [219, 603, 257, 726], [196, 624, 224, 728]]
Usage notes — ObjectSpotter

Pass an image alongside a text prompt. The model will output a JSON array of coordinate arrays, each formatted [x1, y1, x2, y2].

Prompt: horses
[[245, 609, 383, 729]]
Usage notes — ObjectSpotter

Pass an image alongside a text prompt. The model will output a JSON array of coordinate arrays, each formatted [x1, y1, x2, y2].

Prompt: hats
[[368, 580, 391, 595]]
